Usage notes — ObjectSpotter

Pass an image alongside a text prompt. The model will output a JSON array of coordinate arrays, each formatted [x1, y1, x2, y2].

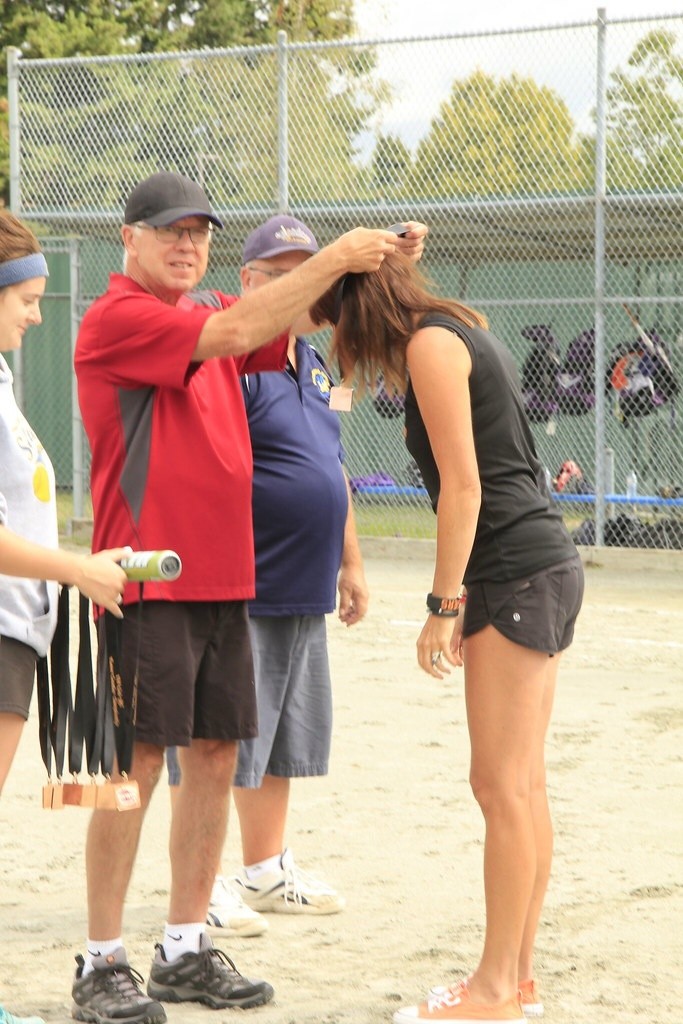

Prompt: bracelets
[[426, 592, 467, 619]]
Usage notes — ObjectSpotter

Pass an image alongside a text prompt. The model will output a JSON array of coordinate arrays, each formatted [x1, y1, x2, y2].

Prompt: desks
[[358, 487, 681, 515]]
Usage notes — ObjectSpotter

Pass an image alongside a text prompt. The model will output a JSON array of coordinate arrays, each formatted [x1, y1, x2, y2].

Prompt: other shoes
[[0, 1005, 45, 1024]]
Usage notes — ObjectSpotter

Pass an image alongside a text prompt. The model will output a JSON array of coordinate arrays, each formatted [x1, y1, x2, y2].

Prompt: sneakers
[[204, 877, 267, 937], [236, 849, 343, 914], [70, 948, 168, 1024], [147, 933, 273, 1008], [393, 978, 544, 1024]]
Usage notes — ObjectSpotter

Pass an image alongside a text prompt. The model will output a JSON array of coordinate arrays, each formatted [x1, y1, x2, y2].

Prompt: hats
[[126, 171, 223, 230], [241, 216, 319, 264]]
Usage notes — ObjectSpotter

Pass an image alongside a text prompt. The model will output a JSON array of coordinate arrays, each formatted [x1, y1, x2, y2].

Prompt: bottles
[[626, 470, 636, 496]]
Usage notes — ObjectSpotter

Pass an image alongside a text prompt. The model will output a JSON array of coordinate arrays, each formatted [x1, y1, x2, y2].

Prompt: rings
[[431, 652, 440, 666]]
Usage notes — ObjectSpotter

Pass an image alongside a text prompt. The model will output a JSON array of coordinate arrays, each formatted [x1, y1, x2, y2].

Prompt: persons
[[166, 214, 367, 937], [308, 246, 585, 1024], [73, 172, 429, 1024], [0, 205, 132, 795]]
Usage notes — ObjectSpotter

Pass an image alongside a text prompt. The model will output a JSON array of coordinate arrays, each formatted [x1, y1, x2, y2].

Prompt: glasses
[[248, 267, 291, 280], [133, 222, 215, 243]]
